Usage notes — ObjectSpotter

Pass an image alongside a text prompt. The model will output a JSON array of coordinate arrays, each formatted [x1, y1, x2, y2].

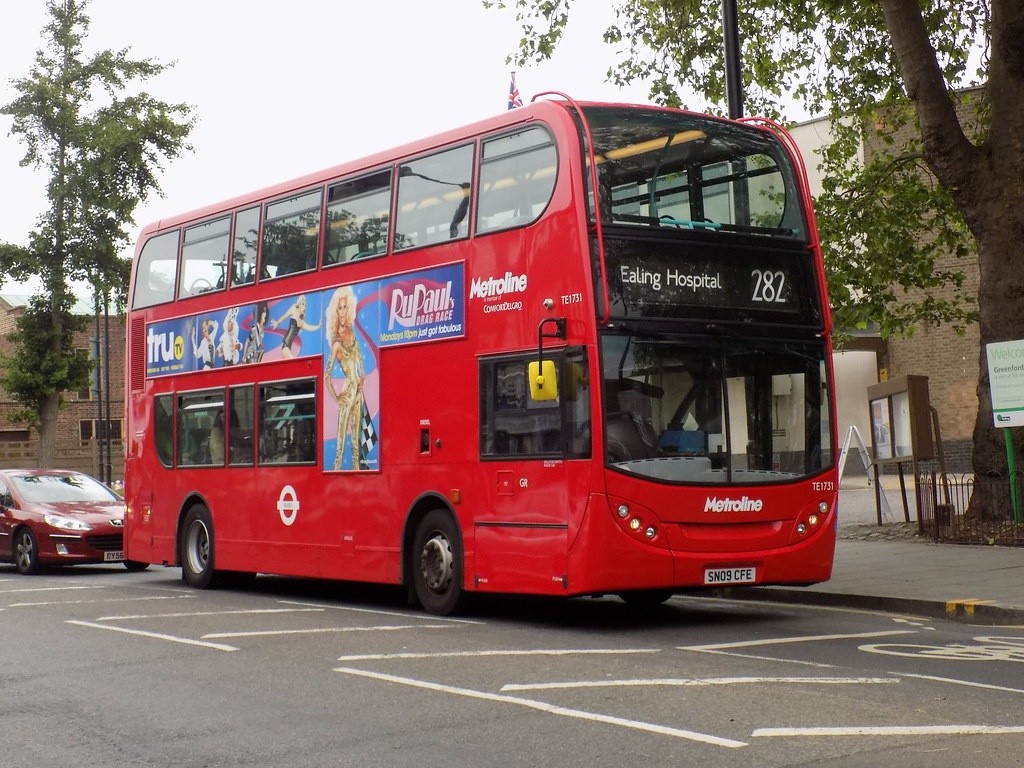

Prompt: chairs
[[583, 412, 656, 462], [190, 251, 381, 294], [660, 214, 680, 228], [199, 435, 212, 464], [694, 218, 719, 232]]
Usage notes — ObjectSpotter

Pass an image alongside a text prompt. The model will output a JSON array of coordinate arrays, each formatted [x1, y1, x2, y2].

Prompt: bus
[[121, 91, 840, 617]]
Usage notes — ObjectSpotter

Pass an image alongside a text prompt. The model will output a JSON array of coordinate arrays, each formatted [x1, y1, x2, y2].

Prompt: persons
[[209, 409, 247, 464], [241, 301, 269, 364], [188, 317, 221, 373], [323, 284, 367, 471], [215, 308, 243, 364], [271, 295, 325, 360], [159, 413, 198, 466]]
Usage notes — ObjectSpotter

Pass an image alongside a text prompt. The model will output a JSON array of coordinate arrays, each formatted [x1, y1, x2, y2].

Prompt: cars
[[0, 468, 150, 576]]
